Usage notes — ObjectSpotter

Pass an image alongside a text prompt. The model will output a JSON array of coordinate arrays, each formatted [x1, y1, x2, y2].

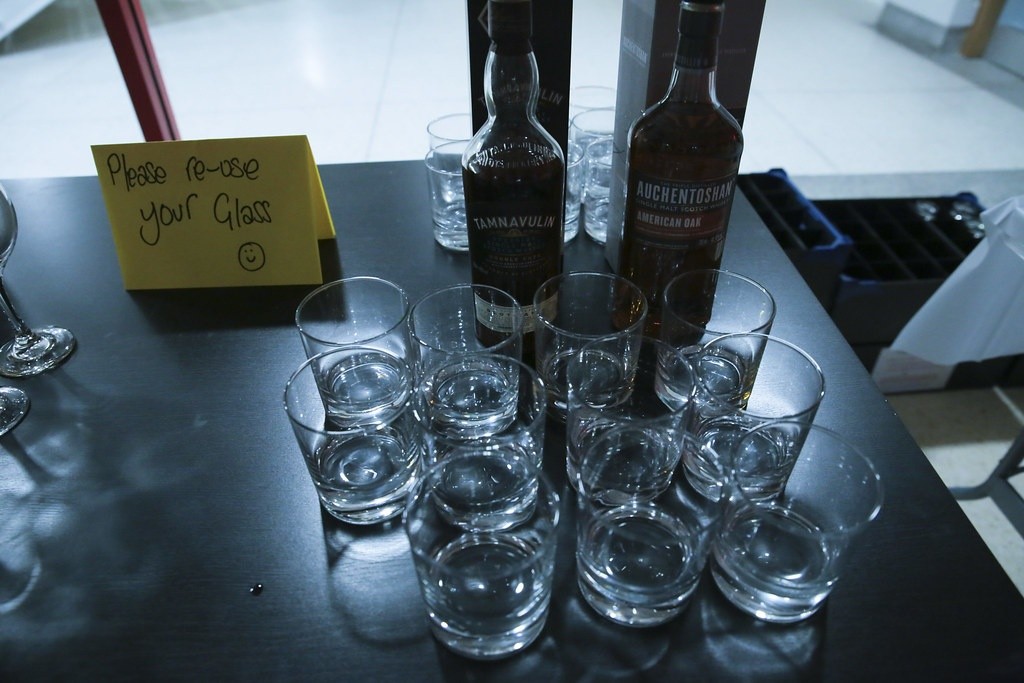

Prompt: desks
[[0, 162, 1024, 683]]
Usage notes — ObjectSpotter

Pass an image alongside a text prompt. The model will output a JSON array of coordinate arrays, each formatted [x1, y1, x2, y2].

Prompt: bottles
[[610, 0, 744, 346], [460, 0, 566, 366]]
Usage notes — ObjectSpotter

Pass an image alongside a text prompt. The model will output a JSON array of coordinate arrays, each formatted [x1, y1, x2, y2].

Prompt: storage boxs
[[738, 168, 1024, 391]]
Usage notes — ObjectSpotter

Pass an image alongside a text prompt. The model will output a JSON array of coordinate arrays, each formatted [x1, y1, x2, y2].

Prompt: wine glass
[[0, 184, 75, 378]]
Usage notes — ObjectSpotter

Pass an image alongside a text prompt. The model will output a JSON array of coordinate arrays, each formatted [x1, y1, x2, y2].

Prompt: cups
[[284, 267, 886, 660], [424, 83, 625, 250]]
